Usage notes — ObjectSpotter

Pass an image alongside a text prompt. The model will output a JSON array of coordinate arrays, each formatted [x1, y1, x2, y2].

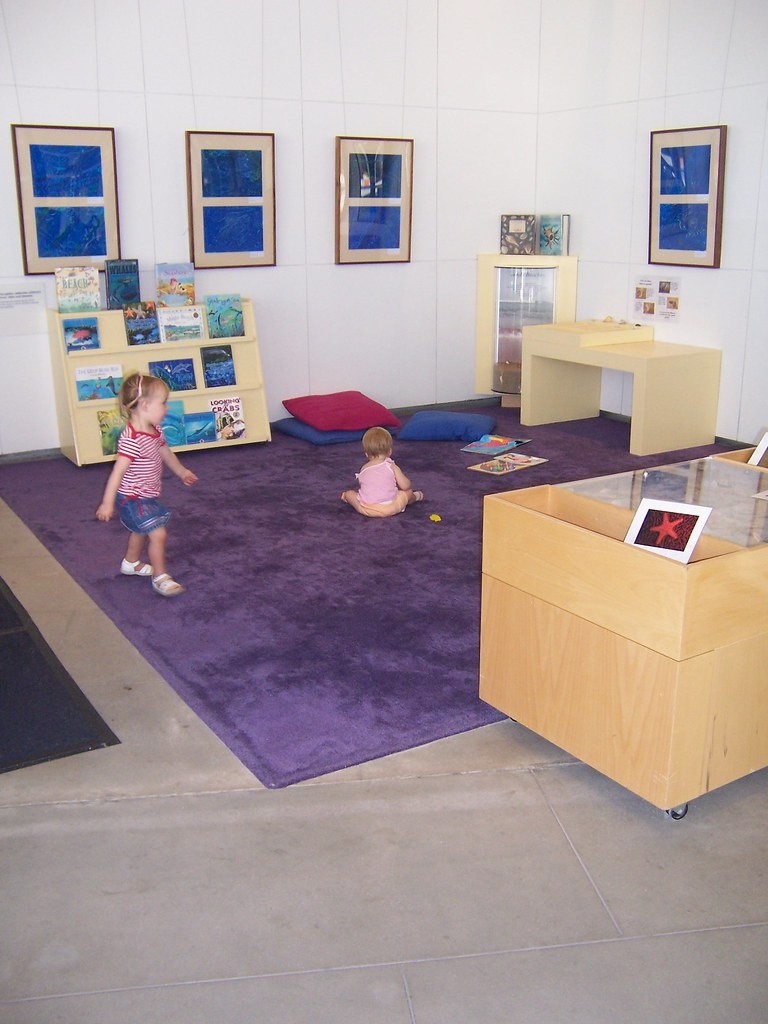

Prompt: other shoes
[[341, 492, 348, 503], [413, 491, 424, 501]]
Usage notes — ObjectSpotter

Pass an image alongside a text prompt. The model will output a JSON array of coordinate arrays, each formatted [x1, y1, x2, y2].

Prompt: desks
[[520, 318, 723, 457]]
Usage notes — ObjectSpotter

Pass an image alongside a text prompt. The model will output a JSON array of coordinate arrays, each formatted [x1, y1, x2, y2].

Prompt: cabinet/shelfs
[[47, 300, 273, 468], [475, 254, 579, 408], [478, 448, 768, 820]]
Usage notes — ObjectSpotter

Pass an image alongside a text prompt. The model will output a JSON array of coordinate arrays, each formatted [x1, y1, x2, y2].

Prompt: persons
[[96, 374, 199, 598], [340, 426, 424, 518]]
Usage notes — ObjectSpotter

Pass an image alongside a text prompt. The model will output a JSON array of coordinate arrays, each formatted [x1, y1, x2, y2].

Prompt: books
[[162, 400, 187, 447], [98, 408, 130, 456], [184, 411, 216, 444], [468, 452, 549, 476], [205, 293, 245, 338], [105, 258, 140, 310], [124, 301, 160, 344], [208, 397, 247, 440], [64, 317, 98, 352], [55, 266, 99, 313], [154, 262, 195, 307], [200, 345, 236, 388], [149, 359, 197, 391], [76, 363, 123, 401], [460, 433, 531, 457], [156, 306, 205, 343]]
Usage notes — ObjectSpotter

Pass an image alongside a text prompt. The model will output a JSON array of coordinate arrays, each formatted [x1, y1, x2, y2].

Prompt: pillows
[[282, 391, 404, 432], [396, 410, 497, 442], [271, 418, 399, 446]]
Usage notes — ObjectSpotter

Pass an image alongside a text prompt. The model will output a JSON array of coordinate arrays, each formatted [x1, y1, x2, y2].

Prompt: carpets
[[0, 396, 739, 791]]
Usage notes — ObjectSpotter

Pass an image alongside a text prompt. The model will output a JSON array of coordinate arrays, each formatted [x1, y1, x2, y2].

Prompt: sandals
[[151, 573, 185, 597], [120, 558, 153, 576]]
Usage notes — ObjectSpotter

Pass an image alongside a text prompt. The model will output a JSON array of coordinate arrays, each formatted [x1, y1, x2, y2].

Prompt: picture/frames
[[648, 124, 728, 269], [335, 136, 414, 264], [185, 130, 277, 270], [10, 123, 122, 276]]
